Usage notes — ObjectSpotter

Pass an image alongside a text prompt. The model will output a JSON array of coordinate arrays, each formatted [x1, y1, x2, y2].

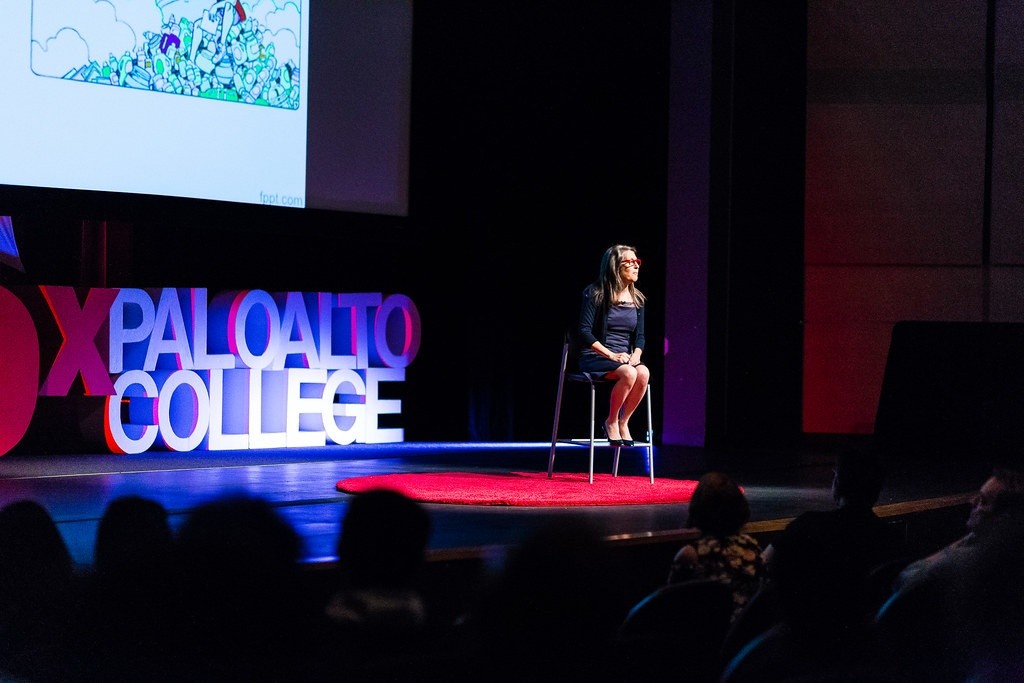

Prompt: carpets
[[334, 468, 744, 508]]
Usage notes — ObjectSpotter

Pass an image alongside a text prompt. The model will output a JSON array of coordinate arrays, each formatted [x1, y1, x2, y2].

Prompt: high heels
[[621, 433, 634, 447], [602, 424, 624, 447]]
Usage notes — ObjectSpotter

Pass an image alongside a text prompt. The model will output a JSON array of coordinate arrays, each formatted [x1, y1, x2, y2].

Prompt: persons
[[665, 471, 762, 610], [764, 439, 893, 579], [897, 469, 1023, 588], [568, 246, 651, 447], [0, 487, 621, 683]]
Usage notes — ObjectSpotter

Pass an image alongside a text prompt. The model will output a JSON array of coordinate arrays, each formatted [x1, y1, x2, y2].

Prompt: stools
[[546, 331, 654, 483]]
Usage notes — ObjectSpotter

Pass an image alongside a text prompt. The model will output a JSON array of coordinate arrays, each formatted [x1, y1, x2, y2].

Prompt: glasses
[[622, 259, 641, 267]]
